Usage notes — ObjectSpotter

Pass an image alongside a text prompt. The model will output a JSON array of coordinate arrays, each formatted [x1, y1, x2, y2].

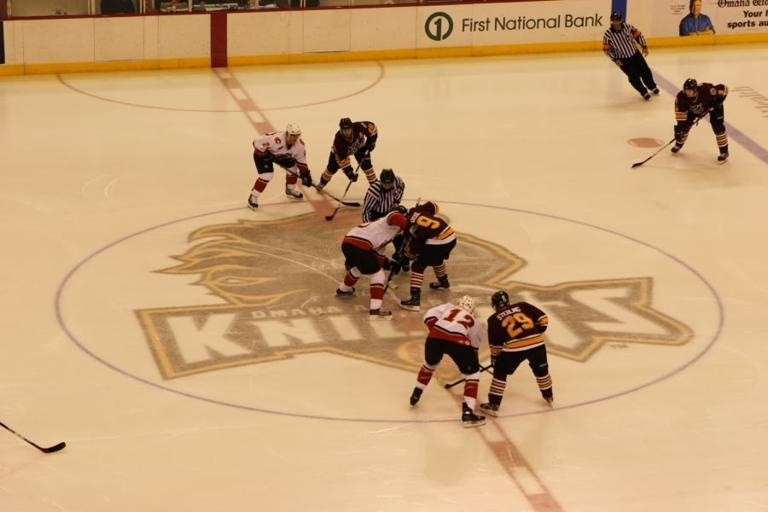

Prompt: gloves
[[348, 171, 357, 181], [300, 172, 312, 187]]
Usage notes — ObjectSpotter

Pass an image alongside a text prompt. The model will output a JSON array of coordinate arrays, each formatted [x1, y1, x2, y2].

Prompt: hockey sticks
[[286, 168, 361, 207], [326, 158, 363, 221], [632, 118, 699, 168], [444, 364, 491, 393], [1, 421, 67, 454]]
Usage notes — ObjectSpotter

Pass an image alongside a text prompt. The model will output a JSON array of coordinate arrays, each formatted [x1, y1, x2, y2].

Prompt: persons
[[247, 123, 312, 208], [316, 116, 380, 188], [481, 289, 556, 411], [360, 167, 411, 273], [396, 201, 456, 303], [403, 296, 488, 426], [335, 205, 405, 319], [678, 0, 714, 36], [670, 77, 730, 160], [602, 11, 660, 101]]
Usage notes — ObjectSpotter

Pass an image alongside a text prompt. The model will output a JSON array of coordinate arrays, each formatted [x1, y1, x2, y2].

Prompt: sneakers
[[672, 141, 683, 152], [542, 390, 553, 399], [248, 193, 257, 207], [643, 87, 659, 99], [462, 403, 499, 420], [718, 147, 729, 160], [286, 183, 302, 197], [336, 255, 449, 317], [411, 387, 422, 405]]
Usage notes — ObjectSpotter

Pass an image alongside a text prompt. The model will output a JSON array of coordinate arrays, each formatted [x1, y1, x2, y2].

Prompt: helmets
[[380, 169, 394, 182], [340, 118, 352, 128], [611, 11, 622, 21], [456, 291, 509, 313], [684, 79, 698, 90], [286, 121, 302, 140]]
